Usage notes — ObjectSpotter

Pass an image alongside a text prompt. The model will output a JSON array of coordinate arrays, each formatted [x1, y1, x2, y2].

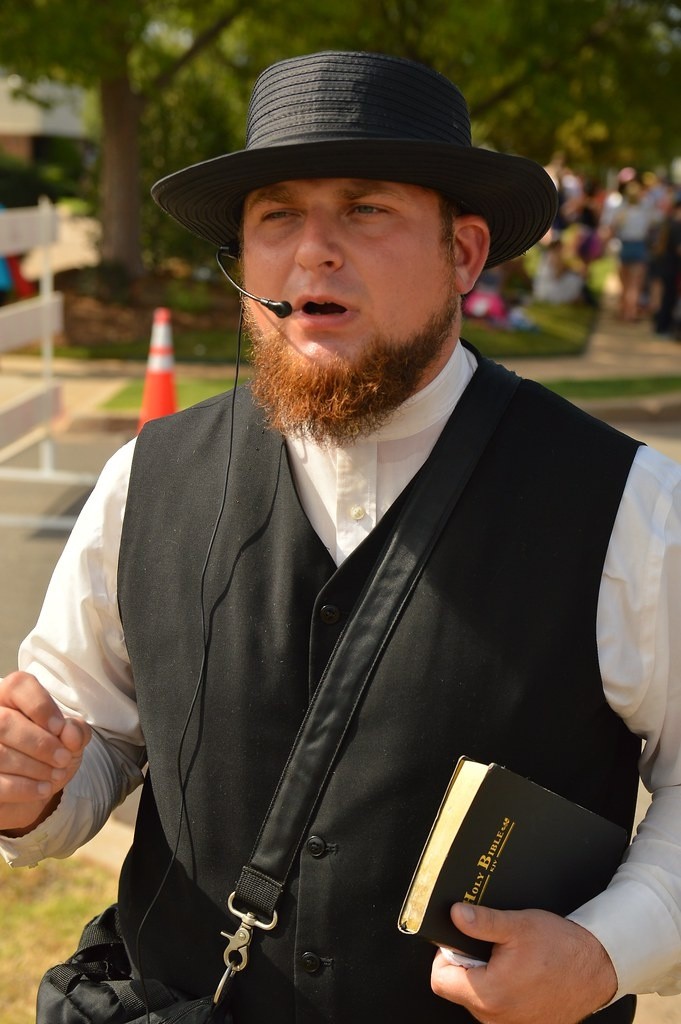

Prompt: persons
[[0, 50, 681, 1024], [460, 161, 680, 342]]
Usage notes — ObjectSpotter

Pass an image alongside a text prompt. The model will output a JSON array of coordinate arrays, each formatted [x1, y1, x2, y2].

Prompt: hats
[[151, 52, 557, 270]]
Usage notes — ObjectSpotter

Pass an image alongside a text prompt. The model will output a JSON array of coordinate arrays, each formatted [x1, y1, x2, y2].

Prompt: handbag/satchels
[[36, 903, 214, 1024]]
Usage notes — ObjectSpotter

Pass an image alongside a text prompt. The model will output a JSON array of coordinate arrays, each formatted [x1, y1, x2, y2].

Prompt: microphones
[[216, 237, 292, 318]]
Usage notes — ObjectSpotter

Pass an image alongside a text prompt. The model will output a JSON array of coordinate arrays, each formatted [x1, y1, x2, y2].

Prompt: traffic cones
[[134, 306, 179, 440]]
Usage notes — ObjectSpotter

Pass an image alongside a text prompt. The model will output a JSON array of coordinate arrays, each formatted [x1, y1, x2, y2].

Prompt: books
[[397, 753, 629, 964]]
